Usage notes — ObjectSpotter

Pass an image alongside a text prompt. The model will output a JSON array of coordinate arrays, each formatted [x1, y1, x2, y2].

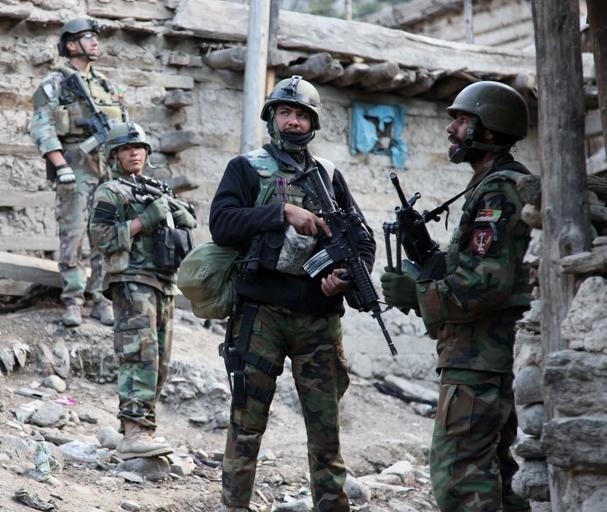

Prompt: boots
[[62, 304, 82, 325], [90, 298, 115, 324], [115, 417, 174, 460]]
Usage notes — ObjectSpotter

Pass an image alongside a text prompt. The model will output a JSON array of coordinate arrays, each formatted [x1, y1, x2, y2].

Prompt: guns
[[61, 72, 111, 153], [287, 166, 399, 357], [118, 173, 196, 228], [383, 172, 440, 316]]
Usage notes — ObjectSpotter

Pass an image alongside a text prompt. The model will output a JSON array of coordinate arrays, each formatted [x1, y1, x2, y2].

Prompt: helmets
[[260, 74, 322, 130], [103, 122, 152, 163], [445, 81, 530, 139], [57, 17, 100, 57]]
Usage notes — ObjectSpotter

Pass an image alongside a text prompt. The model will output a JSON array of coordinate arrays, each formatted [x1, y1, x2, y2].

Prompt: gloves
[[57, 164, 77, 191], [380, 266, 417, 306], [169, 199, 196, 228], [138, 200, 167, 229]]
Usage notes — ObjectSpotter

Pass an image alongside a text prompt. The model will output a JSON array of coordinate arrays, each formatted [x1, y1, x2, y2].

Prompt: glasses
[[70, 33, 99, 42]]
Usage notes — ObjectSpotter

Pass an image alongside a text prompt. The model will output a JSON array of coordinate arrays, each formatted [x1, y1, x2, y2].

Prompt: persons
[[208, 73, 377, 512], [378, 79, 534, 512], [27, 15, 131, 328], [88, 118, 198, 461]]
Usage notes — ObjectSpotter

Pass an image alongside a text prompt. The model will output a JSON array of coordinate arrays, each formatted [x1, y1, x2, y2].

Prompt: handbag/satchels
[[177, 238, 240, 320]]
